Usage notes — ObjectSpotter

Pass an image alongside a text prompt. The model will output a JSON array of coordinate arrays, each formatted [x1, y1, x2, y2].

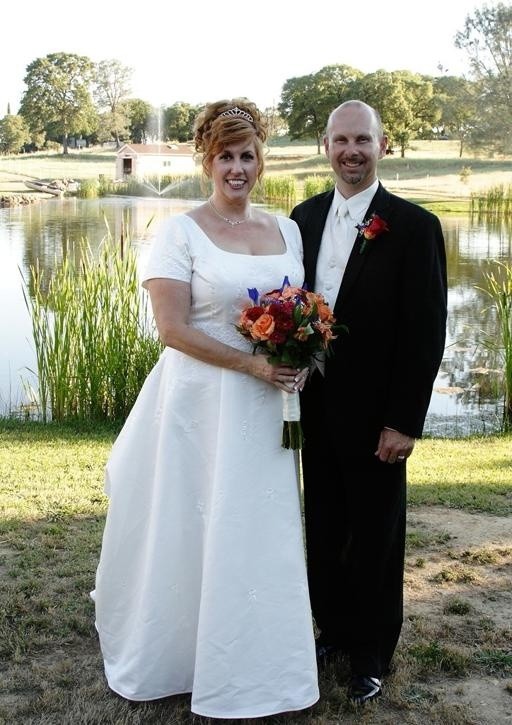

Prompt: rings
[[398, 456, 405, 459]]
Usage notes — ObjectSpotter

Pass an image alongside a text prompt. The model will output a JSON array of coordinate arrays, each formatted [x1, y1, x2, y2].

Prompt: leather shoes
[[347, 673, 383, 705], [314, 632, 338, 657]]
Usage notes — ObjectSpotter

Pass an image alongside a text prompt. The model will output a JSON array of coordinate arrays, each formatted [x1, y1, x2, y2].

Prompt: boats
[[24, 176, 83, 198]]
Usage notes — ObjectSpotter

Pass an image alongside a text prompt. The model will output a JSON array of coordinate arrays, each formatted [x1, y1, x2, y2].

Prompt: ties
[[331, 204, 350, 248]]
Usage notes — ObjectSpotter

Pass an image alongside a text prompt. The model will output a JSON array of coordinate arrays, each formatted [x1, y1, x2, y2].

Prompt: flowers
[[354, 213, 390, 256], [230, 272, 347, 450]]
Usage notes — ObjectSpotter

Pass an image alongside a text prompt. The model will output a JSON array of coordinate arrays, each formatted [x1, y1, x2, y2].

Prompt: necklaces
[[206, 196, 252, 227]]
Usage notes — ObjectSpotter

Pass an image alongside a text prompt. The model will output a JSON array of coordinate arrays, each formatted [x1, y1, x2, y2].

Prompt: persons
[[89, 97, 321, 719], [290, 98, 448, 709]]
